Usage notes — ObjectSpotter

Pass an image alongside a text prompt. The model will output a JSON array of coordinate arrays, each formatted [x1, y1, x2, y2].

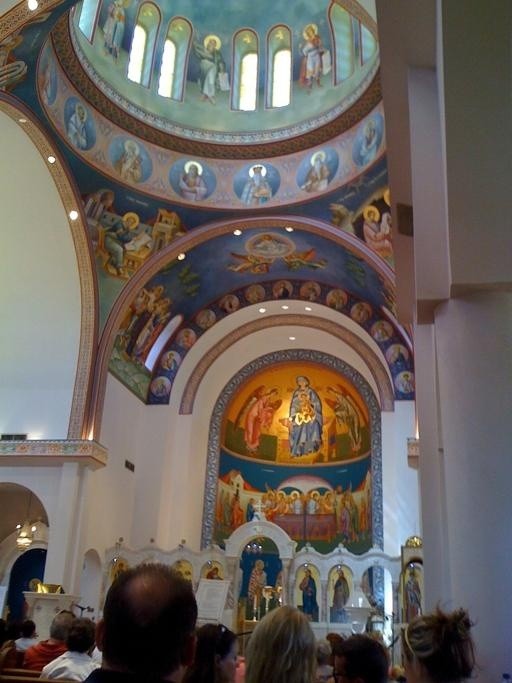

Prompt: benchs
[[0, 668, 83, 682]]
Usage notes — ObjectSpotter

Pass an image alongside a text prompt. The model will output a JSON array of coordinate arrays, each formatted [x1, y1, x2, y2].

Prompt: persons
[[103, 209, 140, 276], [0, 610, 102, 682], [234, 382, 282, 454], [297, 23, 329, 94], [83, 565, 477, 683], [192, 39, 228, 104], [322, 383, 369, 450], [284, 375, 324, 458], [103, 0, 126, 63], [361, 202, 393, 256]]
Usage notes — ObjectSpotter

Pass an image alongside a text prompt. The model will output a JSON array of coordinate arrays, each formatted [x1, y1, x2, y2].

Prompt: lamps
[[16, 492, 37, 552]]
